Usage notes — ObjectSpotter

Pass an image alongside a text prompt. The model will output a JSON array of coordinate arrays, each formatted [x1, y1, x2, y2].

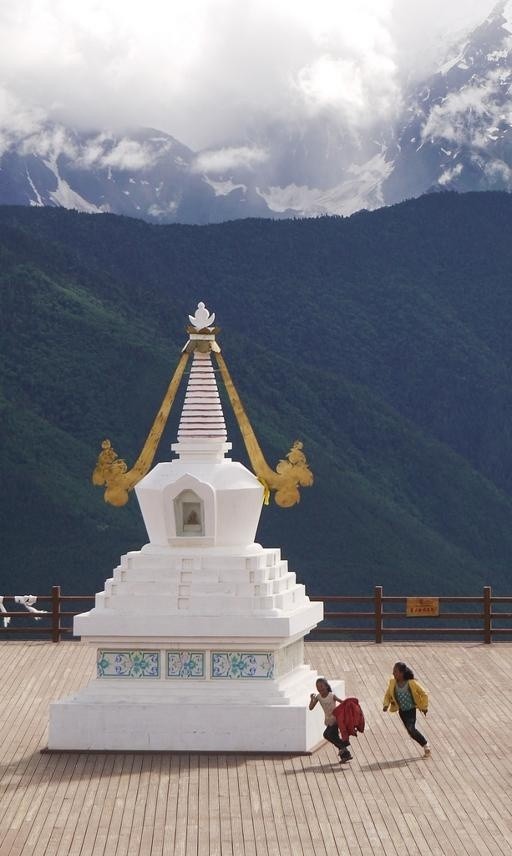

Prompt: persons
[[383, 661, 432, 760], [309, 678, 353, 763]]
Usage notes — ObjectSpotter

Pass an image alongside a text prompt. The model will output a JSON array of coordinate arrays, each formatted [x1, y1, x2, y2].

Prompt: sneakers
[[338, 747, 346, 755], [424, 746, 430, 756], [339, 756, 353, 763]]
